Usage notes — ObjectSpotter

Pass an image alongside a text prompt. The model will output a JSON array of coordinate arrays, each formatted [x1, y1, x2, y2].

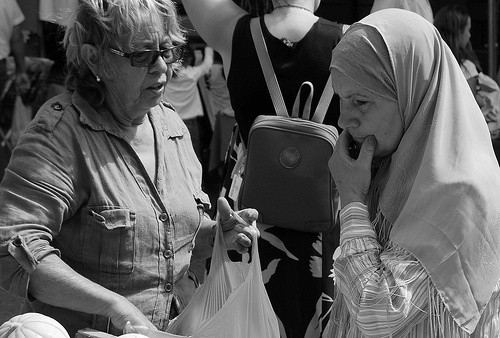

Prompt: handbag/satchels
[[305, 293, 334, 338], [123, 208, 280, 338], [9, 95, 32, 147]]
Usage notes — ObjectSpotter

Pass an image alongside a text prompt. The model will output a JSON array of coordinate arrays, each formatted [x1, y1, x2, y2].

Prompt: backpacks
[[238, 17, 350, 232]]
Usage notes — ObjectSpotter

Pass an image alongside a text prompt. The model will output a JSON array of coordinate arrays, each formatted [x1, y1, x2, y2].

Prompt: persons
[[0, 0, 500, 338]]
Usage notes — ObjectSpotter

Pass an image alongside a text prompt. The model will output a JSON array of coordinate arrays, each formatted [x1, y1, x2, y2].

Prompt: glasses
[[103, 44, 184, 68]]
